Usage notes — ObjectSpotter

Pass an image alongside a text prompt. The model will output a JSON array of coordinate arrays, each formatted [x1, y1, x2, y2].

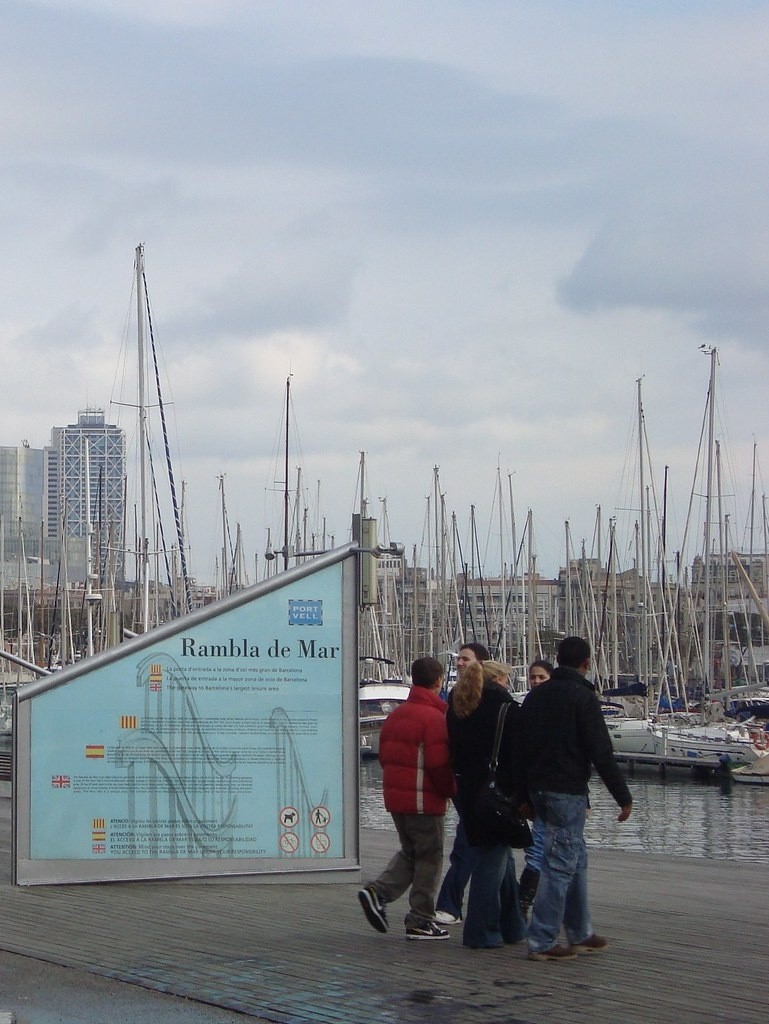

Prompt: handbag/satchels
[[462, 703, 536, 849]]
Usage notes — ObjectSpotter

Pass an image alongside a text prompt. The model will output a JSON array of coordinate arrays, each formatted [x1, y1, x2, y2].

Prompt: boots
[[518, 868, 540, 923]]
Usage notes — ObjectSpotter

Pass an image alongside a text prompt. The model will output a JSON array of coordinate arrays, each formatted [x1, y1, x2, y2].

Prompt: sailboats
[[0, 243, 768, 783]]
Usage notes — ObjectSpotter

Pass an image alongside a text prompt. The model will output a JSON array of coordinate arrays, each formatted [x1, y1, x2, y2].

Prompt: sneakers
[[406, 922, 450, 940], [528, 944, 577, 962], [358, 886, 390, 934], [568, 934, 610, 955], [433, 909, 464, 924]]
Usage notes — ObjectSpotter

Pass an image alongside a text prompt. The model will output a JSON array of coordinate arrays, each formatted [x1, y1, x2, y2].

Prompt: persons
[[435, 644, 490, 925], [519, 635, 633, 959], [358, 657, 450, 939], [452, 660, 529, 946], [519, 660, 552, 921]]
